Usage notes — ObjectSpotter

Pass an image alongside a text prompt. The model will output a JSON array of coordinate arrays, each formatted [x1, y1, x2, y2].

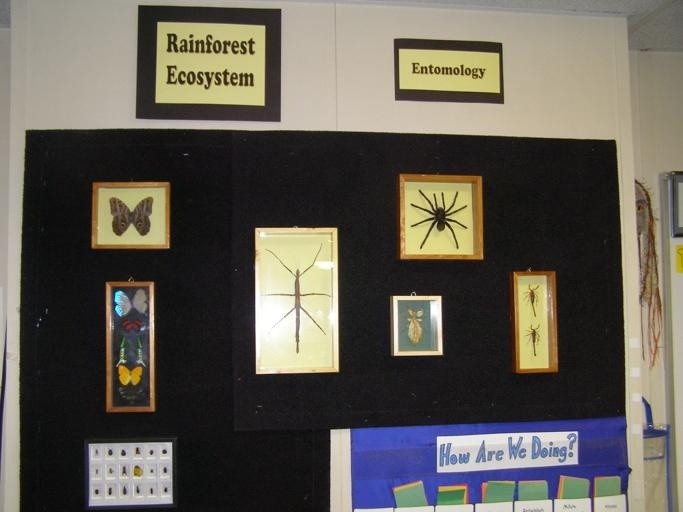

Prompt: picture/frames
[[390, 294, 444, 359], [397, 172, 484, 262], [513, 271, 559, 373], [92, 181, 170, 252], [82, 436, 177, 511], [254, 226, 341, 378], [103, 281, 154, 412]]
